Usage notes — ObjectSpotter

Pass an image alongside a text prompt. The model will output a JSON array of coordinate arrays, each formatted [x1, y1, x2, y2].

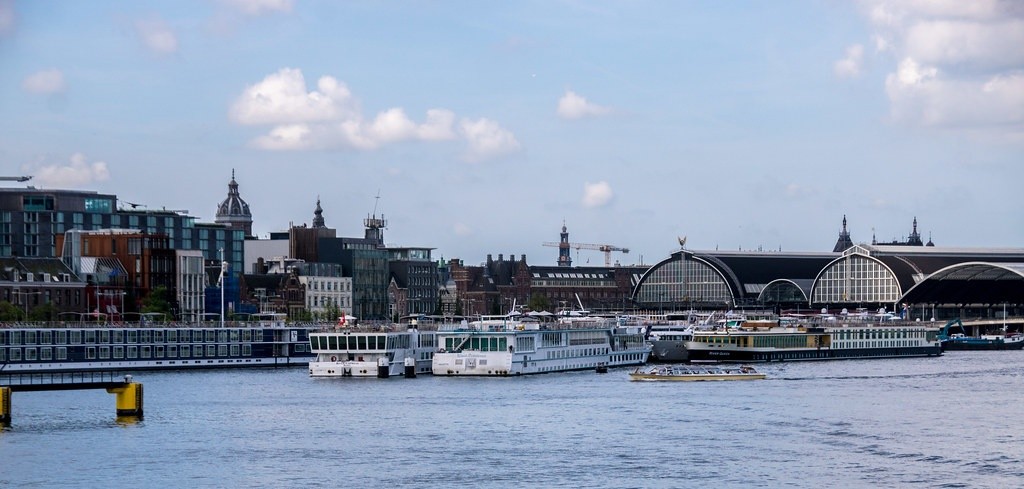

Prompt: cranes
[[541, 241, 630, 266]]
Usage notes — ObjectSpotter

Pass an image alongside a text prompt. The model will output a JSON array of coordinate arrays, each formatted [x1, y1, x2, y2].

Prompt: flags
[[339, 311, 346, 327], [106, 266, 119, 277]]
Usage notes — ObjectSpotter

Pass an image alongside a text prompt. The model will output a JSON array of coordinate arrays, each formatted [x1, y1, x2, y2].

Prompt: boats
[[684, 325, 943, 366], [308, 331, 437, 377], [652, 330, 691, 363], [628, 364, 767, 381], [0, 327, 322, 374], [432, 326, 653, 377]]
